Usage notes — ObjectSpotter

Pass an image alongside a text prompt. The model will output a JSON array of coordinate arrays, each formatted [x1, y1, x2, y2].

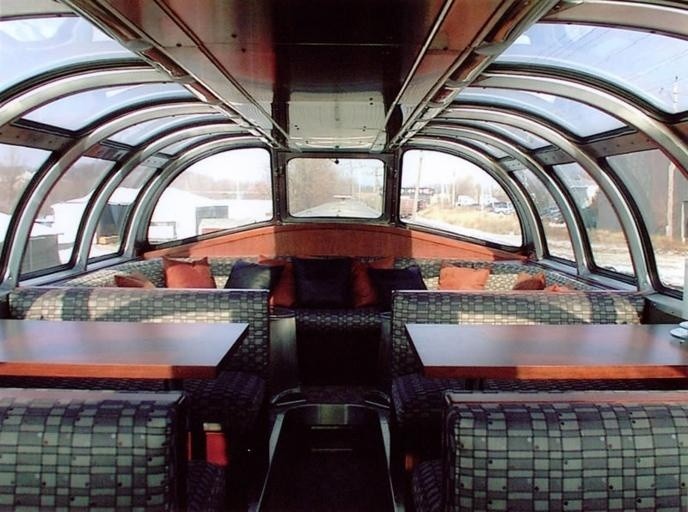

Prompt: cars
[[544, 207, 596, 226], [493, 202, 515, 214]]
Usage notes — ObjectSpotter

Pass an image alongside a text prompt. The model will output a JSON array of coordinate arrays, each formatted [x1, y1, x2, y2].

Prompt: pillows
[[113, 253, 429, 306], [435, 259, 492, 290], [510, 272, 545, 289]]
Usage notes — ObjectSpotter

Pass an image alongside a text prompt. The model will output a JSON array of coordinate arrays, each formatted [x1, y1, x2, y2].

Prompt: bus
[[380, 188, 434, 201]]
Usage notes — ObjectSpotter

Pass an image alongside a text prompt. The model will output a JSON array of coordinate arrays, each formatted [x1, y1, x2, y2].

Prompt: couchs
[[70, 252, 597, 333]]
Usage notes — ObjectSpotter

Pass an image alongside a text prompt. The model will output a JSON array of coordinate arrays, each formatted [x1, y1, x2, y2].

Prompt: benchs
[[3, 287, 267, 508], [387, 290, 685, 510]]
